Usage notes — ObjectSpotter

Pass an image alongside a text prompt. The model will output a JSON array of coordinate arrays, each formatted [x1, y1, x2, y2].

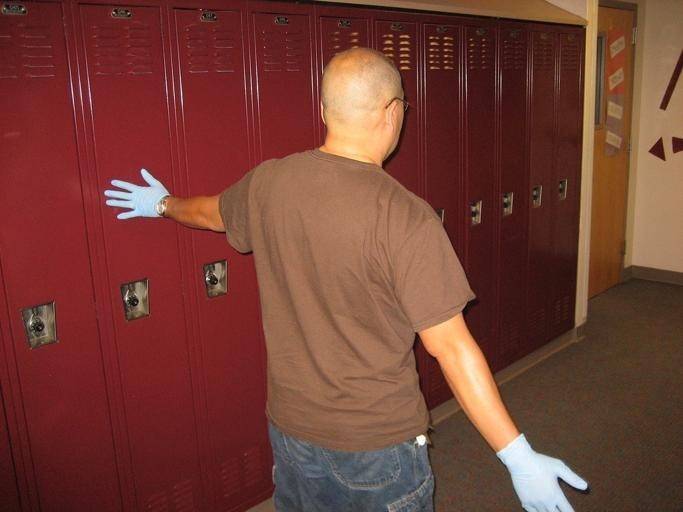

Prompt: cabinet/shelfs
[[0, 1, 316, 512], [318, 4, 587, 406]]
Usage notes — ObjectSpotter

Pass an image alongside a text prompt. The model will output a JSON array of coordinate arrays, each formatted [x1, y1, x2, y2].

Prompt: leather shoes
[[104, 167, 170, 220], [495, 432, 588, 511]]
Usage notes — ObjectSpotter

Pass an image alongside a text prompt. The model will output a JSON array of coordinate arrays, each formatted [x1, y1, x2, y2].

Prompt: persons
[[103, 47, 594, 511]]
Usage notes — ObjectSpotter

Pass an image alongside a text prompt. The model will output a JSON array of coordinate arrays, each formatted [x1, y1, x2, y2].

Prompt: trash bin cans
[[155, 196, 175, 218]]
